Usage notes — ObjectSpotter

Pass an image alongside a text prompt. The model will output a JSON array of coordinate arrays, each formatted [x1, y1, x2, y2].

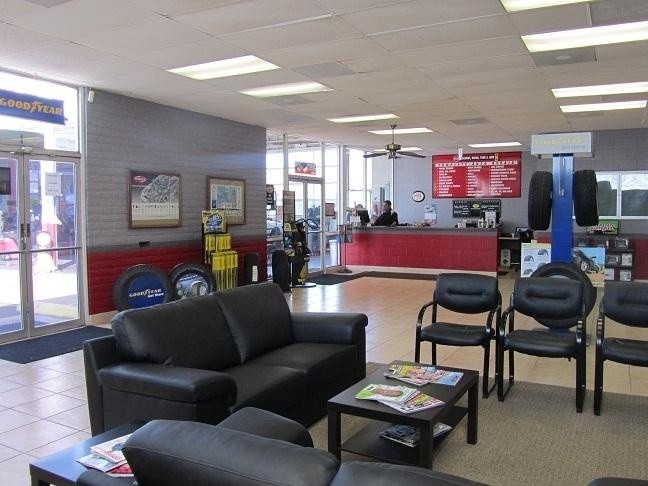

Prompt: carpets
[[1, 324, 114, 364], [305, 273, 354, 286], [307, 358, 648, 486]]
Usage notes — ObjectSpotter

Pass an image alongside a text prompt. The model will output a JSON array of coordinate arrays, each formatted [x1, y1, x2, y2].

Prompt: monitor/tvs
[[358, 210, 370, 228], [480, 211, 499, 229]]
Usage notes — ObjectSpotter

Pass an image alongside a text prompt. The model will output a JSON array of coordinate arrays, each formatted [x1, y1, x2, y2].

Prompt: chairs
[[413, 273, 503, 400], [592, 279, 647, 415], [495, 275, 589, 413]]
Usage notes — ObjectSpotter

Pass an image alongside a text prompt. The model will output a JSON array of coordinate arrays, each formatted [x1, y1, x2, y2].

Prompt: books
[[377, 421, 454, 449], [355, 364, 464, 416], [76, 433, 134, 479]]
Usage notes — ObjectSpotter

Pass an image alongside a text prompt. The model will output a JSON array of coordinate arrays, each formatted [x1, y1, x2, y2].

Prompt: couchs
[[80, 280, 369, 440], [121, 404, 648, 486]]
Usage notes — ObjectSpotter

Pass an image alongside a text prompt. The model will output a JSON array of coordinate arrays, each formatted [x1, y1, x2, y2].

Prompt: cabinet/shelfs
[[497, 232, 521, 273], [575, 232, 638, 284]]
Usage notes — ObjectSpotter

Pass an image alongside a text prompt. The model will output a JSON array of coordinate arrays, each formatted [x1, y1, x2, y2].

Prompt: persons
[[371, 200, 399, 227]]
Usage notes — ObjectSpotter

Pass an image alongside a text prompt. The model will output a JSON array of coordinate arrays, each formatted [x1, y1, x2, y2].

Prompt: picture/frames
[[128, 165, 184, 230], [205, 174, 247, 227]]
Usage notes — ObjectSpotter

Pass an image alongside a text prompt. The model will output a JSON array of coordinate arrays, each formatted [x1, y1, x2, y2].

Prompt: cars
[[266, 219, 289, 266], [290, 217, 338, 256], [0, 209, 41, 242]]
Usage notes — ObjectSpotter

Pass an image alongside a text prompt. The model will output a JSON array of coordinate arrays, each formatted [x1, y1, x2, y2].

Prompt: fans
[[361, 122, 429, 162]]
